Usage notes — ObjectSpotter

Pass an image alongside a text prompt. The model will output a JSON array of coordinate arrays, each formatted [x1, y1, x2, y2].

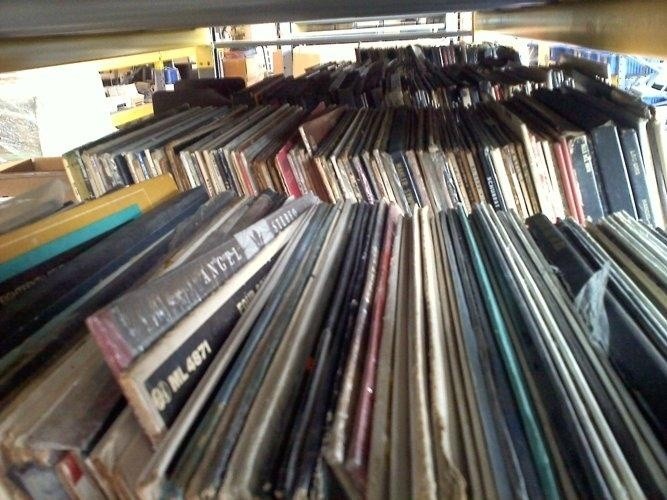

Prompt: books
[[0, 41, 667, 500]]
